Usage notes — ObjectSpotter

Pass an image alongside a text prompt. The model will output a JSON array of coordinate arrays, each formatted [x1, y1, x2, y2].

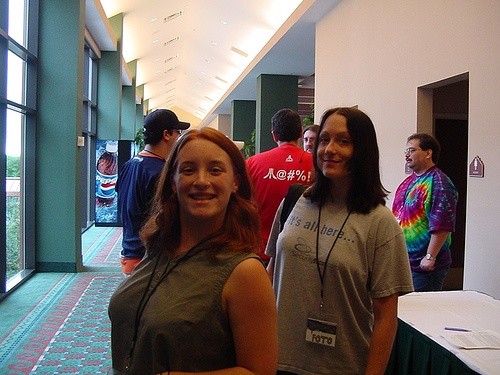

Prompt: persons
[[115, 109, 190, 278], [244, 110, 315, 266], [392, 133, 458, 292], [264, 108, 415, 375], [108, 127, 277, 375], [303, 125, 320, 153]]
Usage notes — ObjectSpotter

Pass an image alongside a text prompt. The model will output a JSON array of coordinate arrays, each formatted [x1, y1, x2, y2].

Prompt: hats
[[142, 108, 191, 135]]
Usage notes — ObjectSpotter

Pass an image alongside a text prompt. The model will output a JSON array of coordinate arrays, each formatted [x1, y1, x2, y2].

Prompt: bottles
[[96, 141, 118, 207]]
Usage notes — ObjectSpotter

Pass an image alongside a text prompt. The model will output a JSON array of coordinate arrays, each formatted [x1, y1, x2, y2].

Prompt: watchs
[[426, 254, 436, 261]]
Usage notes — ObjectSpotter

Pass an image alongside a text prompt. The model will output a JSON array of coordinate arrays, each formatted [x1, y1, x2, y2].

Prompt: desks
[[396, 289, 500, 375]]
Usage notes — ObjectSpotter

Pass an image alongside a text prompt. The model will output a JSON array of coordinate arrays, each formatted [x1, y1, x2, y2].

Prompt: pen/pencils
[[444, 327, 472, 332]]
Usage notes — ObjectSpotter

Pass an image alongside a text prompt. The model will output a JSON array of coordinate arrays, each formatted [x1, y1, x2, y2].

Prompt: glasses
[[405, 148, 422, 154]]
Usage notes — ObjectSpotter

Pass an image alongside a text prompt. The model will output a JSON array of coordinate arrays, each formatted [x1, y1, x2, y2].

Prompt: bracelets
[[160, 371, 170, 375]]
